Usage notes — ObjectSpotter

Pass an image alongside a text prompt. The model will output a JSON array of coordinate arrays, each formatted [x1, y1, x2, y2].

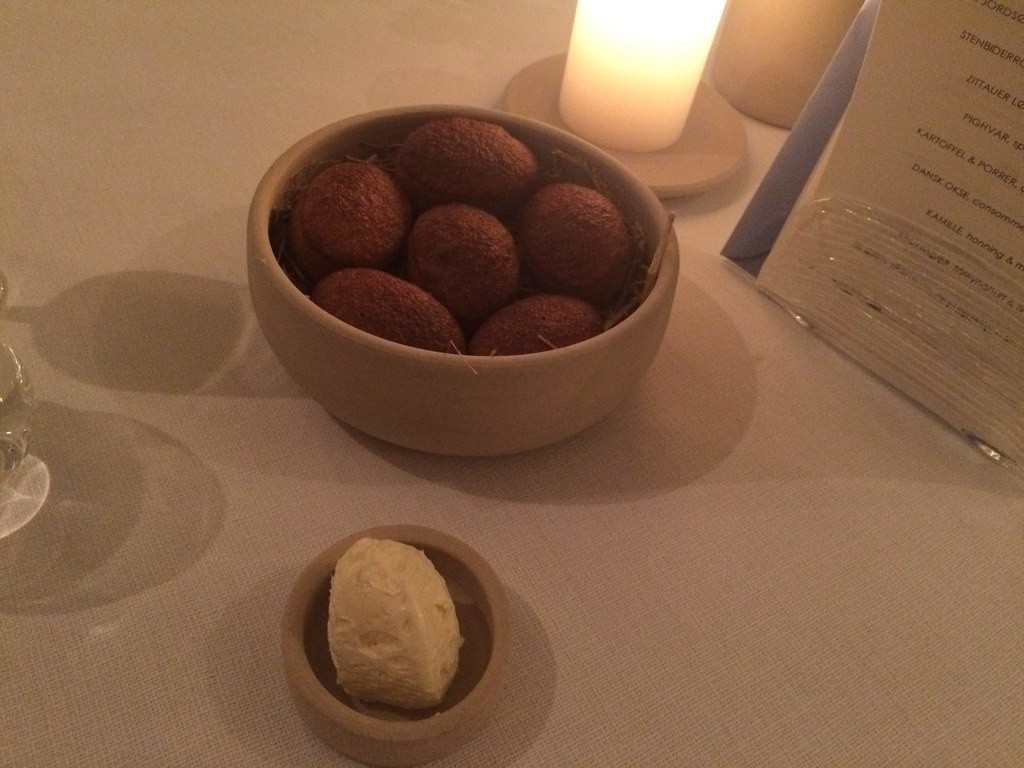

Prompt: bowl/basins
[[281, 525, 512, 768], [247, 103, 680, 458]]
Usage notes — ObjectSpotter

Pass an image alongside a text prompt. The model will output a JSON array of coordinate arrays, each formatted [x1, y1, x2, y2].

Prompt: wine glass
[[0, 342, 49, 540]]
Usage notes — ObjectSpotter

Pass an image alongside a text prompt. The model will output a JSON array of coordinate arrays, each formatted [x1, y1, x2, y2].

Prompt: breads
[[286, 114, 630, 354]]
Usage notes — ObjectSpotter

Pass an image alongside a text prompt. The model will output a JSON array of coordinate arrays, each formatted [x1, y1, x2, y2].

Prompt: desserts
[[326, 536, 464, 709]]
